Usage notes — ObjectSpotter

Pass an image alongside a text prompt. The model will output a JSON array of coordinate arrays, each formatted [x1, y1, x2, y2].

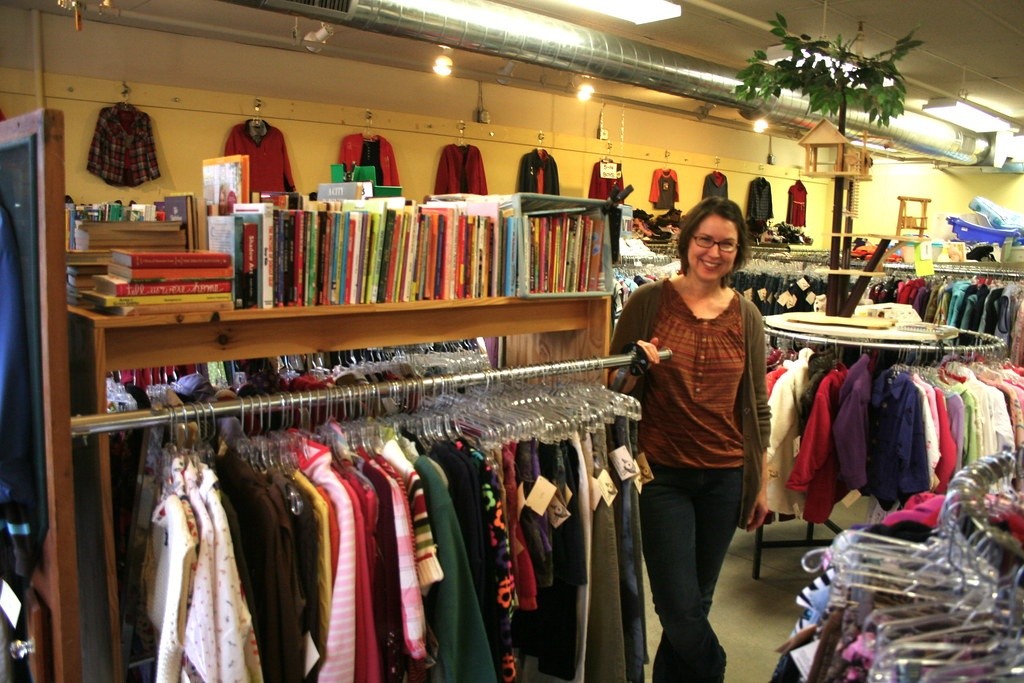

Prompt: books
[[62, 193, 603, 321]]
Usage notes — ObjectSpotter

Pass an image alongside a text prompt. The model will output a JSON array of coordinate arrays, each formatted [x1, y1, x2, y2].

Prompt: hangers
[[604, 245, 1023, 395], [115, 88, 801, 190], [106, 335, 644, 516], [798, 444, 1024, 683]]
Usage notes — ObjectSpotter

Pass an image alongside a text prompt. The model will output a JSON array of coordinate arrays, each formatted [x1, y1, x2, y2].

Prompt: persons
[[217, 185, 229, 215], [607, 194, 773, 683]]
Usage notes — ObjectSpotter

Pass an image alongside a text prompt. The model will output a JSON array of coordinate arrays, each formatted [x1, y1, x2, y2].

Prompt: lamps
[[304, 22, 334, 55]]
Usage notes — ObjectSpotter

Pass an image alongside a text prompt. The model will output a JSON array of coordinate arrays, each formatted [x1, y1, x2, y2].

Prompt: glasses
[[692, 233, 739, 253]]
